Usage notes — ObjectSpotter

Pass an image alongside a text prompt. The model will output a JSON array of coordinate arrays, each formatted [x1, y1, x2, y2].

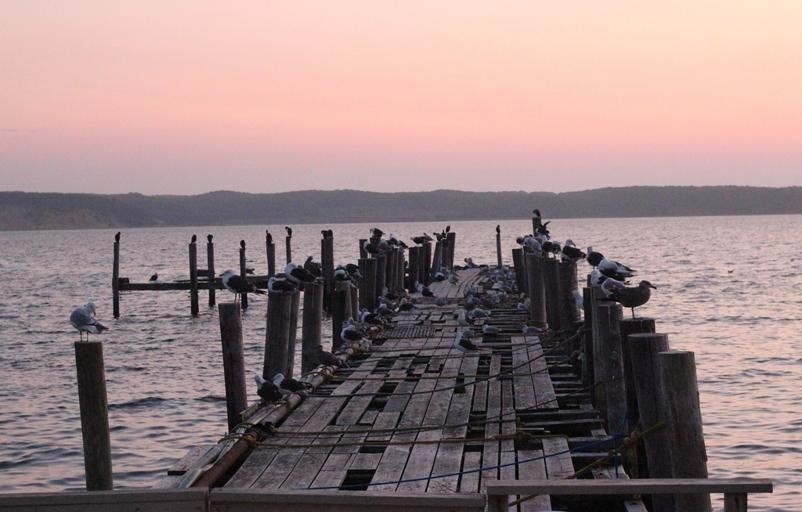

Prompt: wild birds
[[266, 207, 662, 373], [271, 373, 307, 400], [239, 240, 246, 248], [148, 272, 159, 283], [245, 268, 254, 276], [191, 234, 197, 244], [255, 374, 283, 410], [207, 234, 214, 242], [69, 302, 110, 344], [218, 269, 268, 305], [114, 231, 121, 242]]
